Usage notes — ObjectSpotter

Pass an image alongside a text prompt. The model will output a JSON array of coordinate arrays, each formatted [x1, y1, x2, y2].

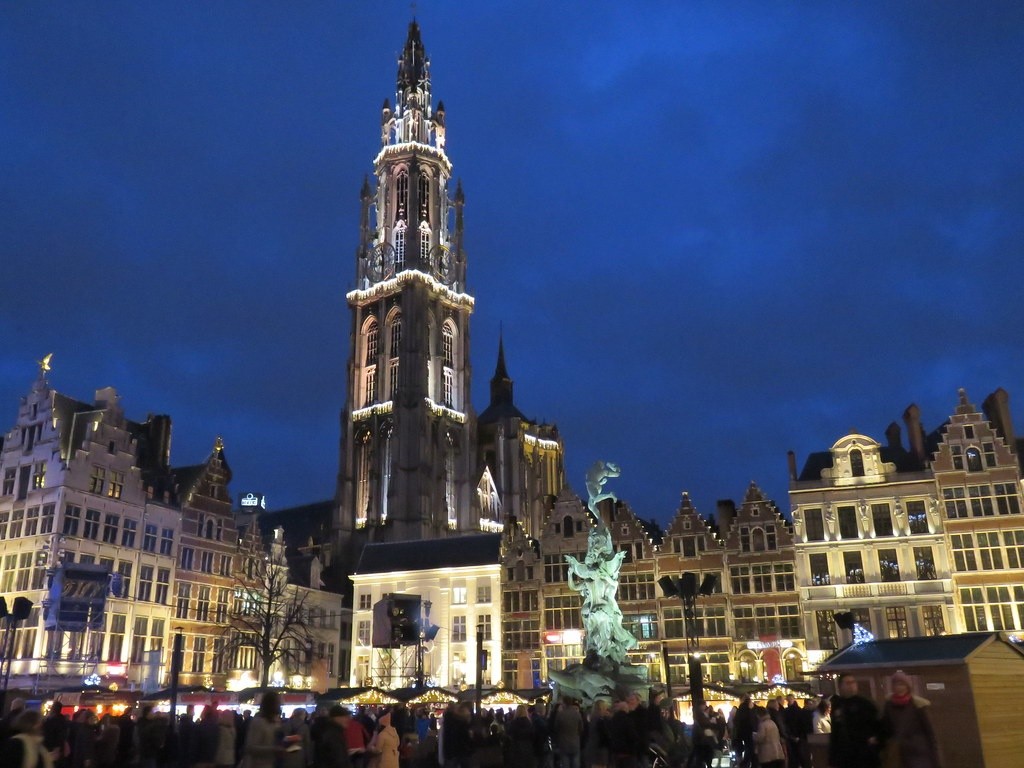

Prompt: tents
[[456, 688, 536, 705], [137, 685, 238, 711], [517, 688, 553, 702], [391, 687, 462, 704], [745, 682, 819, 705], [238, 687, 323, 706], [53, 683, 113, 707], [675, 681, 751, 728], [324, 687, 405, 706]]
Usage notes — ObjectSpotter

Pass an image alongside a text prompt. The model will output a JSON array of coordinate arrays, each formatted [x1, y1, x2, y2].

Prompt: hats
[[890, 669, 912, 690], [329, 706, 351, 718]]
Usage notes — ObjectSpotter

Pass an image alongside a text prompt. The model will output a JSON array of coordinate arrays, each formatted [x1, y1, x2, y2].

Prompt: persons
[[443, 694, 685, 768], [727, 690, 831, 768], [178, 692, 437, 768], [894, 500, 908, 536], [830, 671, 942, 768], [693, 699, 727, 768], [825, 507, 838, 541], [791, 508, 803, 543], [858, 503, 872, 539], [0, 699, 166, 768], [929, 498, 942, 535], [567, 565, 638, 673]]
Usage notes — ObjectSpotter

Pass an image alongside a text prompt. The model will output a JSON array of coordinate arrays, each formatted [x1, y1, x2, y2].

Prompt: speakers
[[657, 572, 717, 597], [0, 596, 9, 618], [428, 625, 439, 640], [833, 612, 855, 629], [12, 597, 34, 619]]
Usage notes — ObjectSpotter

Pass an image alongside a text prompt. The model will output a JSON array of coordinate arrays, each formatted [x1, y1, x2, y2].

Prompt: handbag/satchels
[[878, 738, 903, 768]]
